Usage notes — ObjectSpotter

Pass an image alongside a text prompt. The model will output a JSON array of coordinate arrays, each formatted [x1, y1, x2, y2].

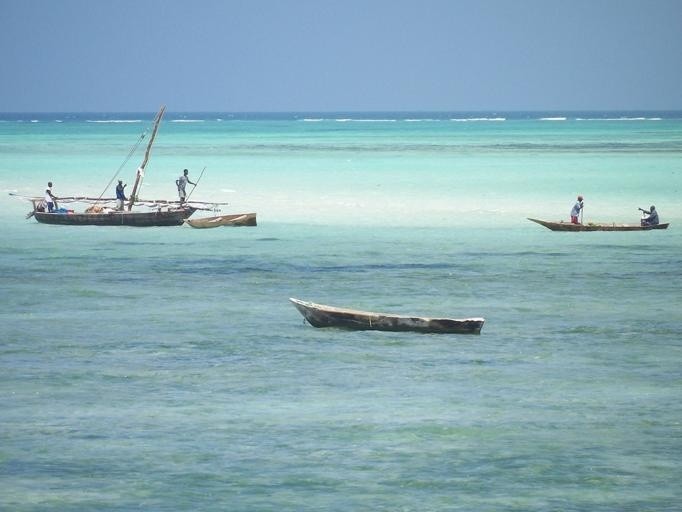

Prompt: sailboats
[[36, 105, 199, 226]]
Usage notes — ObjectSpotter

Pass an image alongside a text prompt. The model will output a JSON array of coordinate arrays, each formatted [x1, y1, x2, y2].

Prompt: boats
[[525, 217, 670, 232], [181, 213, 259, 227], [289, 295, 484, 335]]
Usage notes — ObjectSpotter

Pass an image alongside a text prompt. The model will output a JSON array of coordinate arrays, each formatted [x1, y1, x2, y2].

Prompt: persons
[[45, 181, 58, 212], [175, 169, 196, 203], [570, 194, 584, 224], [637, 204, 661, 226], [116, 177, 129, 210]]
